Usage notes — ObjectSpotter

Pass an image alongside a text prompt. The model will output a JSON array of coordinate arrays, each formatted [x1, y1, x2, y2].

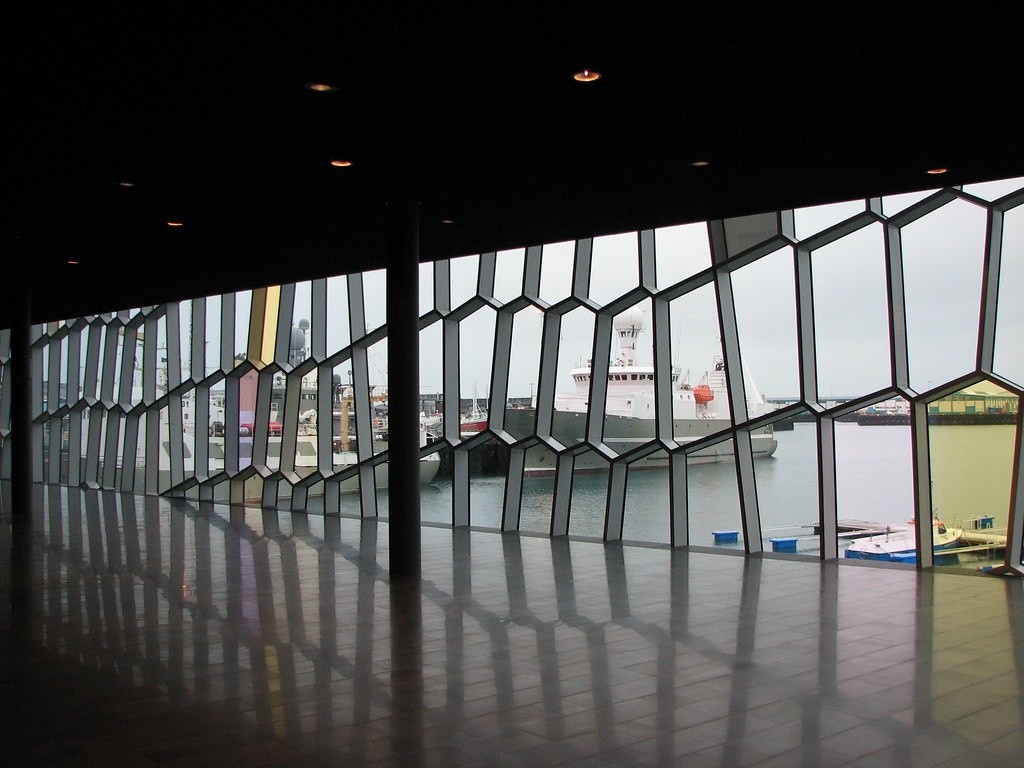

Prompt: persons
[[613, 358, 620, 367]]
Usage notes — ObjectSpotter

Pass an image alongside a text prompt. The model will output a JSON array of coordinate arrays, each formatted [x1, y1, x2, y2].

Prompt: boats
[[43, 327, 511, 504], [844, 516, 964, 565], [504, 306, 778, 478]]
